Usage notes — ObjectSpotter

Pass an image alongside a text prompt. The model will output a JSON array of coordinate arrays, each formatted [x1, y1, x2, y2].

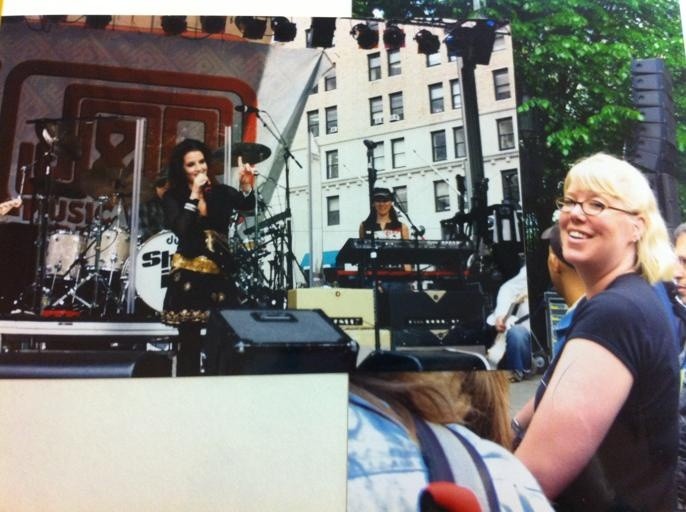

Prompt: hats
[[372, 188, 395, 202]]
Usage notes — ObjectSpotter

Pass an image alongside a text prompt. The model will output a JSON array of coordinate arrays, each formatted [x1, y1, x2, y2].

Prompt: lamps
[[199, 16, 227, 34], [159, 15, 188, 36], [271, 16, 297, 42], [304, 17, 336, 49], [235, 16, 267, 40], [39, 15, 67, 33], [339, 15, 440, 56], [84, 15, 112, 31]]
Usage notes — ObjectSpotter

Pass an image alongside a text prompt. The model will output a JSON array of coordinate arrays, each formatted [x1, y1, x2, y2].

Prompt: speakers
[[641, 173, 681, 247], [198, 308, 360, 376], [625, 58, 678, 175], [356, 348, 491, 373], [0, 349, 156, 379]]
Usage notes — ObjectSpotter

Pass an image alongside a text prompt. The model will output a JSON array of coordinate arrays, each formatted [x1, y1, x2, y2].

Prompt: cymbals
[[85, 168, 158, 205], [210, 142, 271, 173]]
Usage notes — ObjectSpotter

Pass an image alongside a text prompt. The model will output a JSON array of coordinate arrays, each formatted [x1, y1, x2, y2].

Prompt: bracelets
[[511, 417, 525, 440]]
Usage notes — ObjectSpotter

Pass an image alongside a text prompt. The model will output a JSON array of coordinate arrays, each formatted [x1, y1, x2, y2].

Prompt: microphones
[[201, 181, 214, 202], [363, 139, 377, 149], [234, 104, 259, 113]]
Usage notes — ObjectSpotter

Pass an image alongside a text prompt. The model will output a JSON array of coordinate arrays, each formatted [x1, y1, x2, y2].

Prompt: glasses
[[552, 195, 641, 220]]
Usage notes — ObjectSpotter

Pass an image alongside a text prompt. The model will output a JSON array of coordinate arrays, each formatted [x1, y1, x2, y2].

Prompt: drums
[[120, 230, 182, 315], [43, 227, 89, 281], [85, 224, 141, 272], [236, 238, 265, 254]]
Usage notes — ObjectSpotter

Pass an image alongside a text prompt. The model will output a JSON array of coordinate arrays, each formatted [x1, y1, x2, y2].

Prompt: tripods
[[44, 201, 125, 314], [229, 167, 309, 308]]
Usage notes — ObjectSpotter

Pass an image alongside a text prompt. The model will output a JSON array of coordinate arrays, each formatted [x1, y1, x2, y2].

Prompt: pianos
[[335, 237, 474, 272]]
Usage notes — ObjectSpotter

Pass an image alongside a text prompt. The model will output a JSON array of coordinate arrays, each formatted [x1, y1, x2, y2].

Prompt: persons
[[487, 264, 532, 383], [81, 175, 166, 249], [160, 136, 265, 377], [668, 220, 686, 512], [356, 187, 413, 294], [345, 372, 557, 512], [500, 149, 681, 512], [505, 216, 588, 455]]
